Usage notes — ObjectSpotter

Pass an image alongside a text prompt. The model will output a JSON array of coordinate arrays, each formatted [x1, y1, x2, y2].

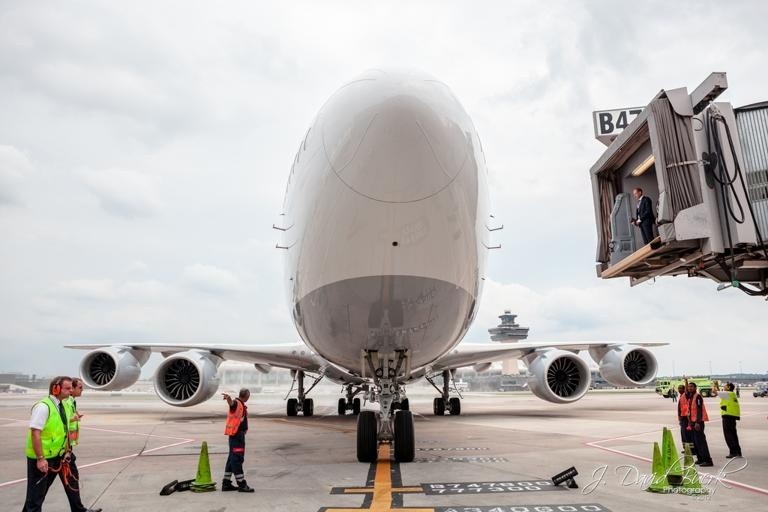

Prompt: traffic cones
[[190, 441, 217, 493], [647, 427, 704, 494]]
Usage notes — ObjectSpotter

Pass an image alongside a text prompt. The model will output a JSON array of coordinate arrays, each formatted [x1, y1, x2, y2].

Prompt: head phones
[[51, 377, 62, 396]]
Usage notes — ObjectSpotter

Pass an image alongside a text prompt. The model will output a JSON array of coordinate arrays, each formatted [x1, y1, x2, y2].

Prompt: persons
[[712, 381, 741, 458], [678, 379, 713, 466], [736, 386, 740, 397], [222, 389, 254, 492], [671, 387, 677, 402], [634, 188, 655, 245], [22, 377, 102, 512]]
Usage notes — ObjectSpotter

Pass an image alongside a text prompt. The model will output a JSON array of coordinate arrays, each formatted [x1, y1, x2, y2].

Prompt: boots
[[222, 479, 254, 492]]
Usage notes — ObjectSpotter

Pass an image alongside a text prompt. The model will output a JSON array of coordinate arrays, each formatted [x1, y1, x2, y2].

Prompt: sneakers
[[694, 457, 713, 466], [726, 453, 742, 458], [681, 451, 685, 454], [85, 508, 102, 512]]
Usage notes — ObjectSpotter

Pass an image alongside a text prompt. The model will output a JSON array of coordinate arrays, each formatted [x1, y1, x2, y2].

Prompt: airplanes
[[61, 76, 669, 463]]
